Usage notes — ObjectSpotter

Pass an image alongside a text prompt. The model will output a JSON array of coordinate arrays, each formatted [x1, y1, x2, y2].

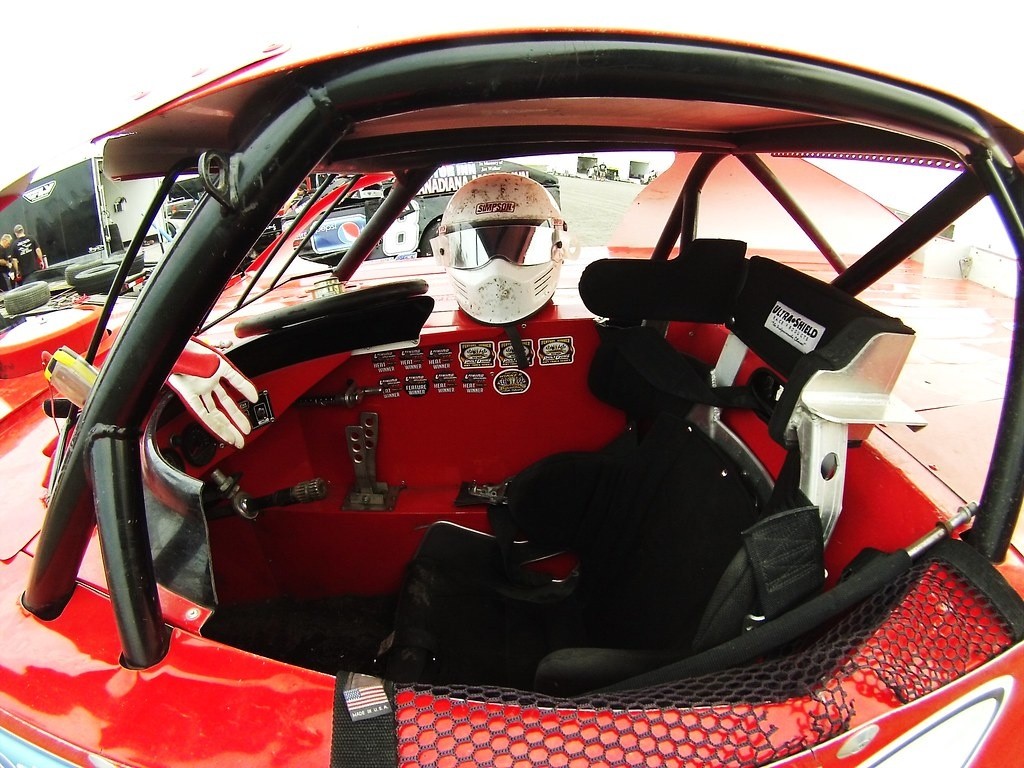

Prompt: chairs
[[370, 238, 928, 707]]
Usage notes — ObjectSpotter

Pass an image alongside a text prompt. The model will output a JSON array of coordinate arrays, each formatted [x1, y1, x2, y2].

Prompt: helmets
[[428, 173, 582, 325]]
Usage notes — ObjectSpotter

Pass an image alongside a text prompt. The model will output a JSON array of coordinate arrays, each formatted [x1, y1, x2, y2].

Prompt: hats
[[13, 224, 24, 233]]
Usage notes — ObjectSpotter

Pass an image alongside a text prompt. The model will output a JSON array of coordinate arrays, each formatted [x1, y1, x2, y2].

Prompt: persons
[[9, 223, 49, 286], [0, 234, 19, 290]]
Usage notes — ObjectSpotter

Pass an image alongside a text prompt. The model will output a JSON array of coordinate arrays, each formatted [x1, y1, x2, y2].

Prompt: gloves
[[164, 335, 261, 450]]
